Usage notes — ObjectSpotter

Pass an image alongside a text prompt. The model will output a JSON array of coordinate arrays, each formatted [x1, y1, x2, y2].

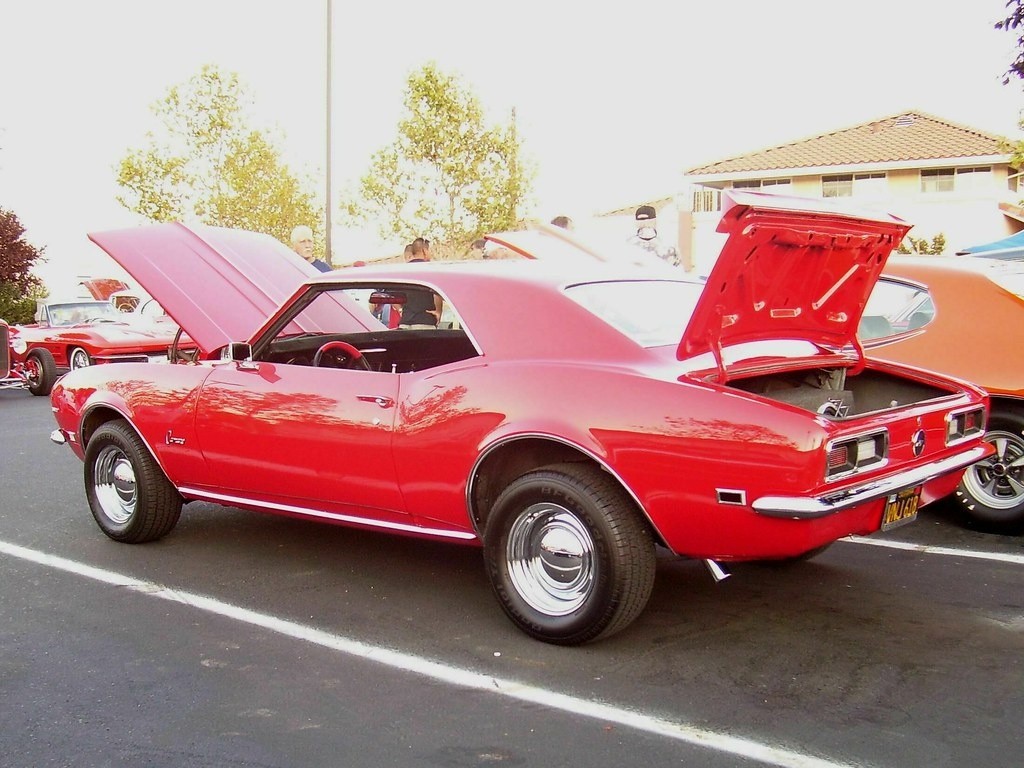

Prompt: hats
[[635, 205, 657, 220]]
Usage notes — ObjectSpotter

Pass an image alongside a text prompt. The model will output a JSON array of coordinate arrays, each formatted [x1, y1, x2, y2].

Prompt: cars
[[856, 250, 1024, 525], [50, 190, 992, 645], [9, 300, 172, 399], [66, 278, 165, 314]]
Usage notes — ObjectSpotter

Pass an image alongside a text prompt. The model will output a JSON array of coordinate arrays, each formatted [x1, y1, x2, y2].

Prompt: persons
[[552, 216, 573, 231], [471, 241, 486, 259], [291, 225, 334, 273], [628, 206, 681, 267], [352, 239, 443, 330]]
